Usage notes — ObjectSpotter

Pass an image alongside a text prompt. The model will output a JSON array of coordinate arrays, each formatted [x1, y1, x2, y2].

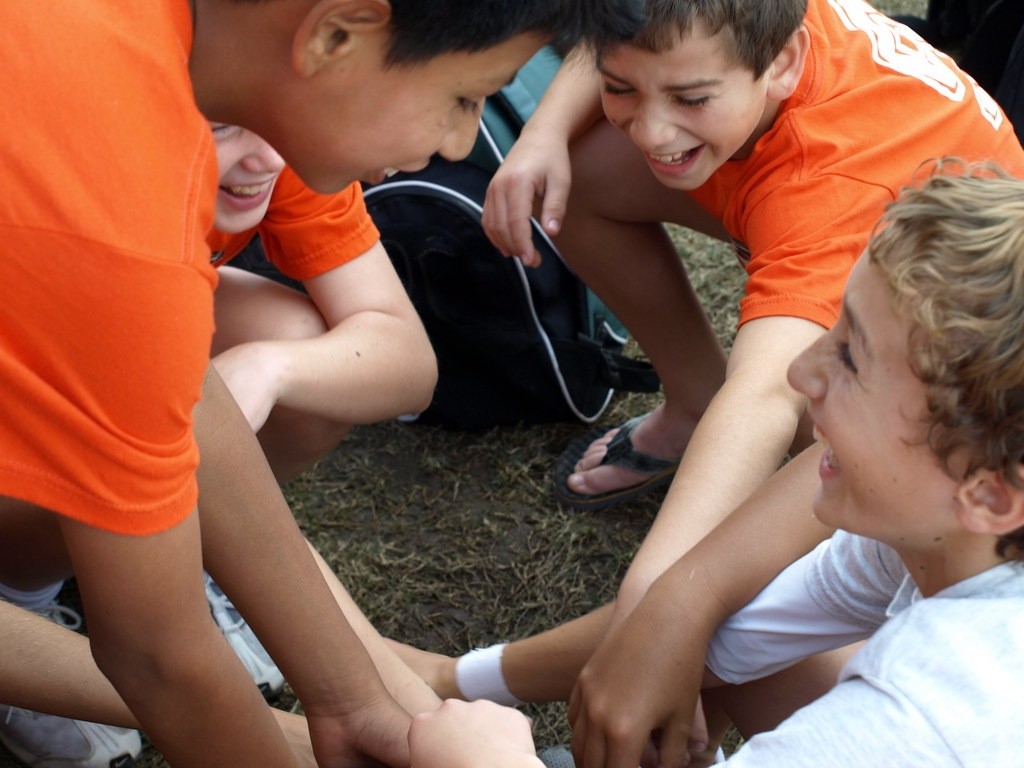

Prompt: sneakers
[[0, 599, 142, 768], [202, 566, 284, 700]]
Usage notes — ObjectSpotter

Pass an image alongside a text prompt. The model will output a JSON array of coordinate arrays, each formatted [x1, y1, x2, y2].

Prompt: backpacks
[[884, 1, 1024, 148], [266, 40, 658, 426]]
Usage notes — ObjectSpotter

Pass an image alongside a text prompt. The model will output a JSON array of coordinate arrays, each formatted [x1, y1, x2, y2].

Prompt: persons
[[0, 0, 1024, 768]]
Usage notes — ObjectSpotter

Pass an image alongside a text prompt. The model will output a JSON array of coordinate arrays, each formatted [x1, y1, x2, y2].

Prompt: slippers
[[554, 410, 681, 510]]
